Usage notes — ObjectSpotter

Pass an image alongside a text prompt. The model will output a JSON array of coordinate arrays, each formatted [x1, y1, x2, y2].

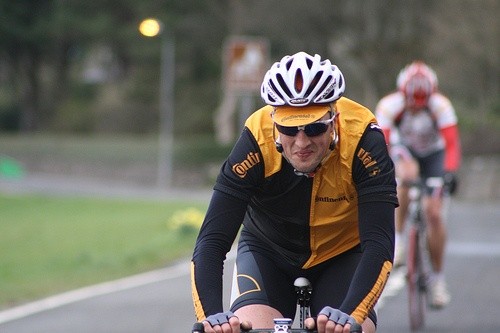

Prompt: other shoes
[[425, 272, 451, 312], [392, 233, 412, 269]]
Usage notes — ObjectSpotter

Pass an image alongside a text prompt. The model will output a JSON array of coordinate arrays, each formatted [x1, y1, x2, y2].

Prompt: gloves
[[441, 171, 459, 197]]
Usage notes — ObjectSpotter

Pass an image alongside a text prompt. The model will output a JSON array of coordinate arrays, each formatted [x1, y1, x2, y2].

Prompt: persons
[[190, 50, 400, 333], [375, 62, 461, 309]]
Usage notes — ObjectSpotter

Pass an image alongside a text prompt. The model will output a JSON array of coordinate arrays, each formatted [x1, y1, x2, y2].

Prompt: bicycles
[[393, 175, 454, 331], [192, 277, 363, 333]]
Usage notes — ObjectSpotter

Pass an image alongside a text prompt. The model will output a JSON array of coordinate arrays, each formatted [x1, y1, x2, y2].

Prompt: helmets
[[397, 60, 440, 110], [259, 51, 347, 106]]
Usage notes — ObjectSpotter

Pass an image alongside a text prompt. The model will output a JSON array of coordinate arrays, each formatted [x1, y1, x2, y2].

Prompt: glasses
[[276, 115, 336, 137]]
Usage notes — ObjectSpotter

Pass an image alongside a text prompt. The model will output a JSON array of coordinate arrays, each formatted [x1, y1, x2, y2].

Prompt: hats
[[272, 77, 338, 127]]
